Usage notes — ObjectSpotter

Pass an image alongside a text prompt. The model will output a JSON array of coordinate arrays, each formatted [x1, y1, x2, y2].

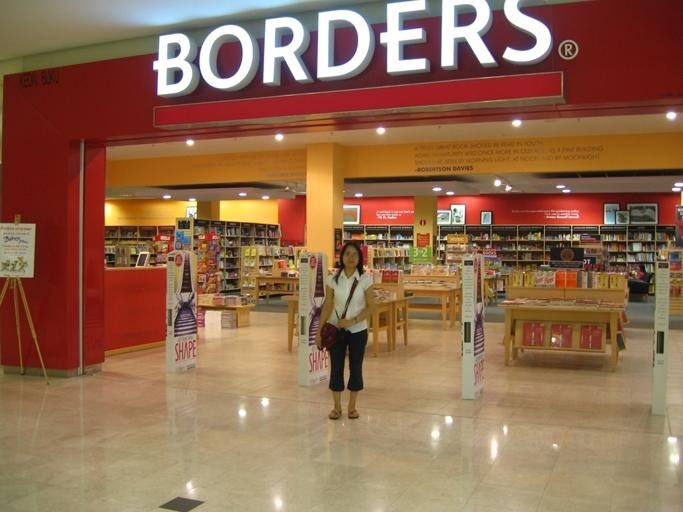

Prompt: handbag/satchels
[[317, 323, 337, 349]]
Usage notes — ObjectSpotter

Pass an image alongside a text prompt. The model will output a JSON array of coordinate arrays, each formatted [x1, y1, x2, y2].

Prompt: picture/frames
[[343, 204, 360, 224], [437, 210, 452, 224], [185, 206, 197, 219], [449, 203, 466, 224], [603, 202, 620, 225], [615, 210, 631, 225], [480, 210, 493, 225], [625, 202, 659, 224]]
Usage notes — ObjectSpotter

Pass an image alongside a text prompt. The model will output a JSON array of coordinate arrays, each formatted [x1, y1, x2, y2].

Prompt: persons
[[315, 243, 377, 420]]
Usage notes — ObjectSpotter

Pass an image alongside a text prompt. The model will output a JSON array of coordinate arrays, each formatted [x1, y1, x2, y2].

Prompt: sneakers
[[328, 410, 341, 419], [348, 410, 359, 418]]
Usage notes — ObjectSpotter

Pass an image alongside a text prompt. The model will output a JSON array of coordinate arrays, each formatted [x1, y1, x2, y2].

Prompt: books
[[105, 223, 683, 353]]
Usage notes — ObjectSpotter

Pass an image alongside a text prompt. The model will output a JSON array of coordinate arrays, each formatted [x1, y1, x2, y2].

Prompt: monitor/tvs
[[136, 251, 151, 267]]
